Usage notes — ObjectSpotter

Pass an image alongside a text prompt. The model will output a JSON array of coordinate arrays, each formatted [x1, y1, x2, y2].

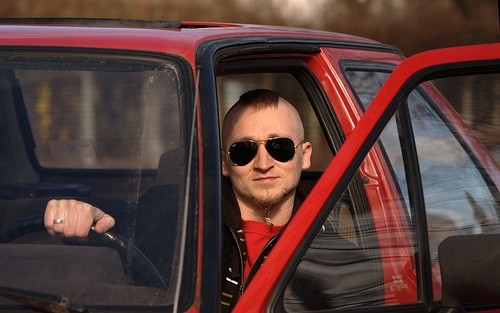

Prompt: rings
[[54, 219, 63, 224]]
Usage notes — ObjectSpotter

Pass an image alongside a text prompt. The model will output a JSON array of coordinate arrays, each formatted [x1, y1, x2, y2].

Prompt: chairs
[[154, 150, 182, 186]]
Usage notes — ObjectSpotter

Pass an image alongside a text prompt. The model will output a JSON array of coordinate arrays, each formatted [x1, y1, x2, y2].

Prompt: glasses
[[221, 137, 305, 166]]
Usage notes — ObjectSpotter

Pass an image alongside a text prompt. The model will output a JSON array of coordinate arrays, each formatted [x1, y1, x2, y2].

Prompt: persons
[[44, 88, 383, 313]]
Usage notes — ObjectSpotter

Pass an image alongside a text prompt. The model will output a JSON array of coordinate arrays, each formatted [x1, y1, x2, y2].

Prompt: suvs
[[0, 17, 2, 313]]
